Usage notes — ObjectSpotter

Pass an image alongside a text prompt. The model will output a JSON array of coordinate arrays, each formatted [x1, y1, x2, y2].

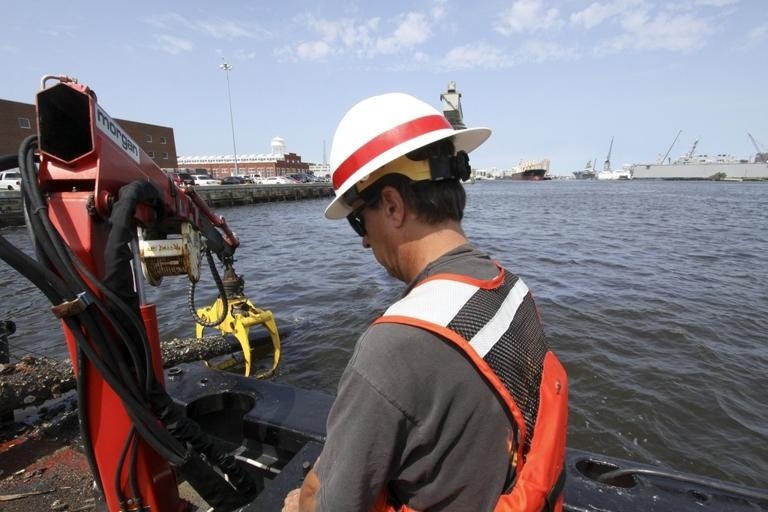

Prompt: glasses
[[346, 196, 378, 236]]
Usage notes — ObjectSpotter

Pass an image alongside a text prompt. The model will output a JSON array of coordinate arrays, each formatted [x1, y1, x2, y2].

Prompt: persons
[[281, 92, 568, 510]]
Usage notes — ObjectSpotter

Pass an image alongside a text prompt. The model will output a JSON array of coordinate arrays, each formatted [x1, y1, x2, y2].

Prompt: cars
[[0, 171, 24, 192], [162, 164, 333, 189]]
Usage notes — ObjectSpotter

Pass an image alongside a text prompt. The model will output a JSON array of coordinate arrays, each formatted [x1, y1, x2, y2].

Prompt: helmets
[[324, 92, 492, 219]]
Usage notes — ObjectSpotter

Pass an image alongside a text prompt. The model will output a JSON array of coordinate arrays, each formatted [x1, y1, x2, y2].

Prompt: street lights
[[219, 61, 240, 177]]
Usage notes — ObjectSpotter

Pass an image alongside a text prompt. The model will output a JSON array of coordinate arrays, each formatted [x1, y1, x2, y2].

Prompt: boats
[[1, 63, 768, 511], [570, 132, 768, 181], [501, 156, 550, 182]]
